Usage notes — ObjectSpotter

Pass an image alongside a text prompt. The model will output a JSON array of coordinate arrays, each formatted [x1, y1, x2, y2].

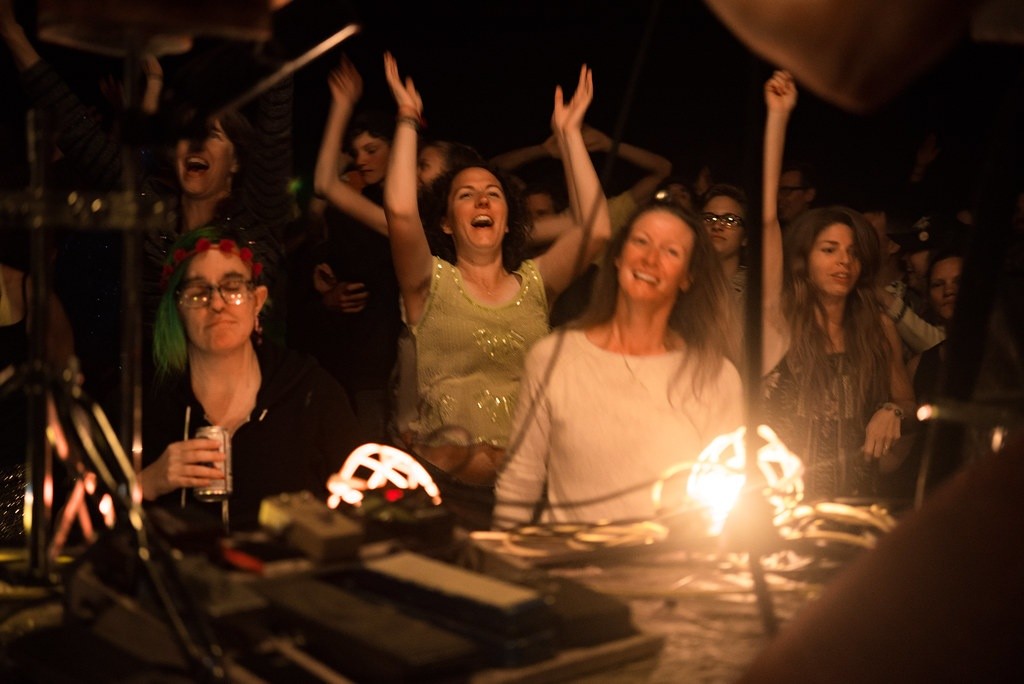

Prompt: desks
[[0, 543, 830, 683]]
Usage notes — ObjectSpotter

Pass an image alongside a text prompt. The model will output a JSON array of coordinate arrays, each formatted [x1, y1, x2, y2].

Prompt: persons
[[0, 0, 966, 511], [383, 51, 615, 529], [0, 262, 83, 551], [493, 198, 745, 632], [132, 229, 361, 530]]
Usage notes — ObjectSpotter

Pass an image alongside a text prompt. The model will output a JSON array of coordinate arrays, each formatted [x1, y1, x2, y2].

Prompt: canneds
[[194, 425, 232, 502]]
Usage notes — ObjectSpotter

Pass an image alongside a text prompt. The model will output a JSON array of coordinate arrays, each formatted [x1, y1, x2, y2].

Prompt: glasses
[[697, 214, 746, 229], [778, 186, 806, 196], [174, 280, 253, 309]]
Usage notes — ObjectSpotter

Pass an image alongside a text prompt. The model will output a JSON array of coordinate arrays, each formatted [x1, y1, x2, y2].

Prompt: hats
[[886, 214, 969, 252]]
